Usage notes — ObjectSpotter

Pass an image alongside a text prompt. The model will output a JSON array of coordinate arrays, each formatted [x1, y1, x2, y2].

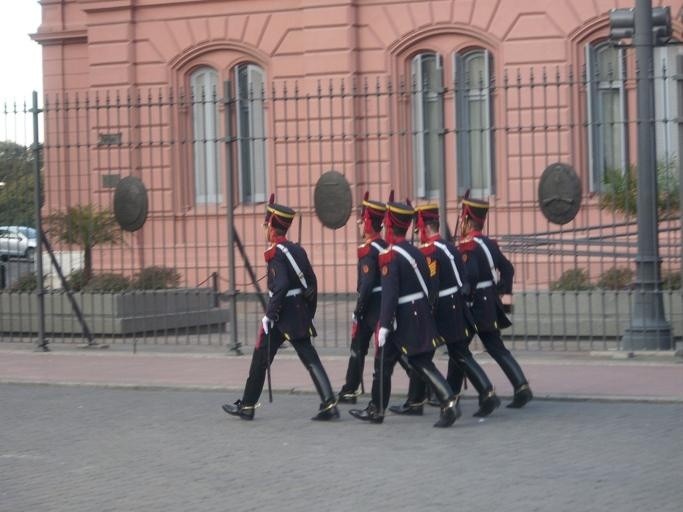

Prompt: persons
[[349, 201, 463, 428], [220, 198, 339, 422], [336, 198, 414, 404], [389, 204, 501, 419], [423, 196, 534, 408]]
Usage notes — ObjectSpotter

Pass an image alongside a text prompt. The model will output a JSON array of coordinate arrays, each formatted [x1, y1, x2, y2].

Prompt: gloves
[[377, 326, 390, 347], [262, 315, 275, 335]]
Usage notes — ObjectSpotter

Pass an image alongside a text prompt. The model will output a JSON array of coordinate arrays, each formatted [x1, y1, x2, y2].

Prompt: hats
[[361, 189, 489, 238], [261, 193, 295, 231]]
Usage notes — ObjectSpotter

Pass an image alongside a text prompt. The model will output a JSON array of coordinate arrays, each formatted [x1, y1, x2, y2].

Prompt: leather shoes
[[348, 400, 386, 424], [473, 389, 499, 417], [310, 398, 340, 421], [506, 382, 533, 408], [433, 396, 462, 428], [333, 385, 358, 403], [223, 399, 255, 420], [389, 398, 425, 415]]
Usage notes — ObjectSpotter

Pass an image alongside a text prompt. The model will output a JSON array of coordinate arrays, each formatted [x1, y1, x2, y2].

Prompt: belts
[[268, 288, 302, 298], [398, 291, 423, 304], [371, 286, 383, 292], [438, 286, 458, 297], [476, 280, 493, 289]]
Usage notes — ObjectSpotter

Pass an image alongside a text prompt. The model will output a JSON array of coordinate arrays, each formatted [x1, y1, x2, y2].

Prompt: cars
[[0, 225, 37, 260]]
